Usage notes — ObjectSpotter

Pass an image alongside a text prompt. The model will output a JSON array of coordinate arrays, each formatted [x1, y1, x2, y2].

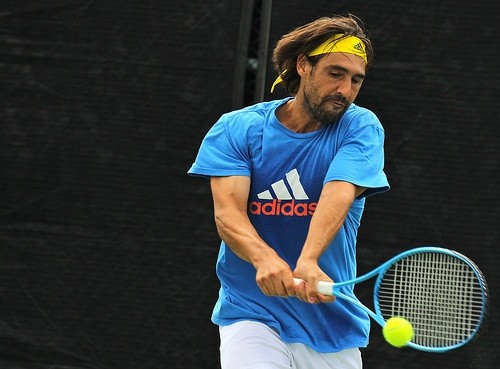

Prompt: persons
[[186, 11, 392, 369]]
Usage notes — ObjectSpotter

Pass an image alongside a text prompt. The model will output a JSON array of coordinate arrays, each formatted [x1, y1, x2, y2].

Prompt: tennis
[[383, 316, 414, 347]]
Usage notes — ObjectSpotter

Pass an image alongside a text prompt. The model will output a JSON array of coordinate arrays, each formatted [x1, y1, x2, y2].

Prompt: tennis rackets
[[293, 247, 488, 354]]
